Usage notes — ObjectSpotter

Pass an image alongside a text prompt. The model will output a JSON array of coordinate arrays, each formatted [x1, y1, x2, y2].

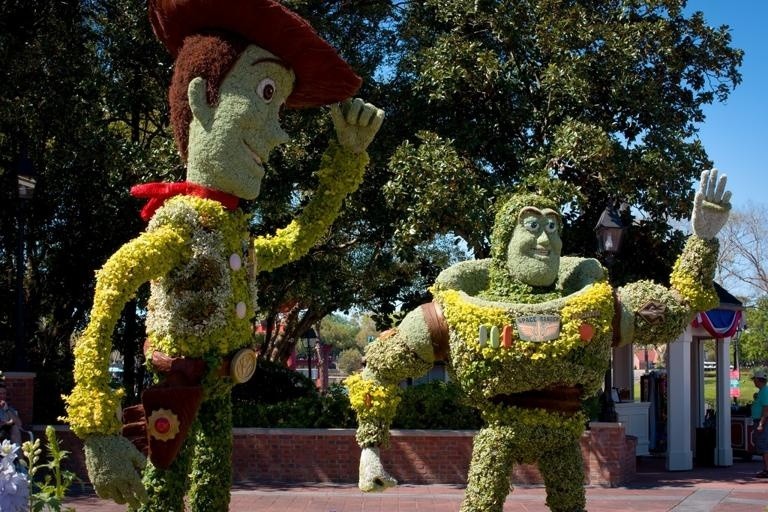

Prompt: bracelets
[[758, 423, 764, 427], [5, 408, 11, 413]]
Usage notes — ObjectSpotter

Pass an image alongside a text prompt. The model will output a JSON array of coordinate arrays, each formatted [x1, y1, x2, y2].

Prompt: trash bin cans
[[612, 402, 652, 456], [648, 361, 653, 369]]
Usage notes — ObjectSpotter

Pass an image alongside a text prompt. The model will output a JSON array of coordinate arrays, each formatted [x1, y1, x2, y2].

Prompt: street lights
[[593, 206, 624, 422], [7, 153, 38, 370]]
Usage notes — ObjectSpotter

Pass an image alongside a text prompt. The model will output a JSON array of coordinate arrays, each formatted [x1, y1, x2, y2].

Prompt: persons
[[345, 163, 732, 512], [0, 384, 24, 464], [750, 367, 768, 478], [59, 1, 386, 512]]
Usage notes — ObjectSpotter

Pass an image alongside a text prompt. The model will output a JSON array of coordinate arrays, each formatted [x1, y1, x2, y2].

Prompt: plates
[[621, 400, 635, 403]]
